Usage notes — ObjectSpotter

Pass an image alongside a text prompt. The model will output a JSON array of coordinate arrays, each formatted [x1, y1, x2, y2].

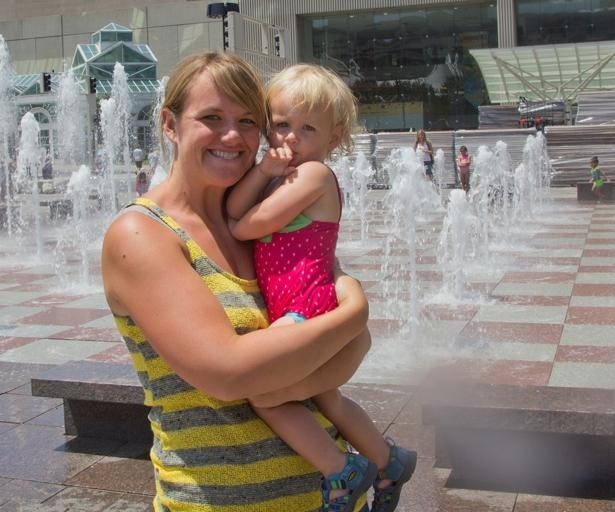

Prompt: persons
[[101, 50, 371, 512], [457, 145, 471, 192], [43, 156, 55, 195], [225, 63, 418, 512], [133, 147, 148, 195], [589, 156, 610, 203], [411, 128, 437, 192]]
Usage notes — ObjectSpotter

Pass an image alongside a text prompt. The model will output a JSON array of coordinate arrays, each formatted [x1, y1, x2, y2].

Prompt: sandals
[[314, 452, 378, 512], [370, 445, 417, 512]]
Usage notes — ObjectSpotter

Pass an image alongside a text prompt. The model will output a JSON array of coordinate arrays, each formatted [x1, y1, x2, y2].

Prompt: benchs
[[421, 379, 615, 485], [32, 360, 151, 443]]
